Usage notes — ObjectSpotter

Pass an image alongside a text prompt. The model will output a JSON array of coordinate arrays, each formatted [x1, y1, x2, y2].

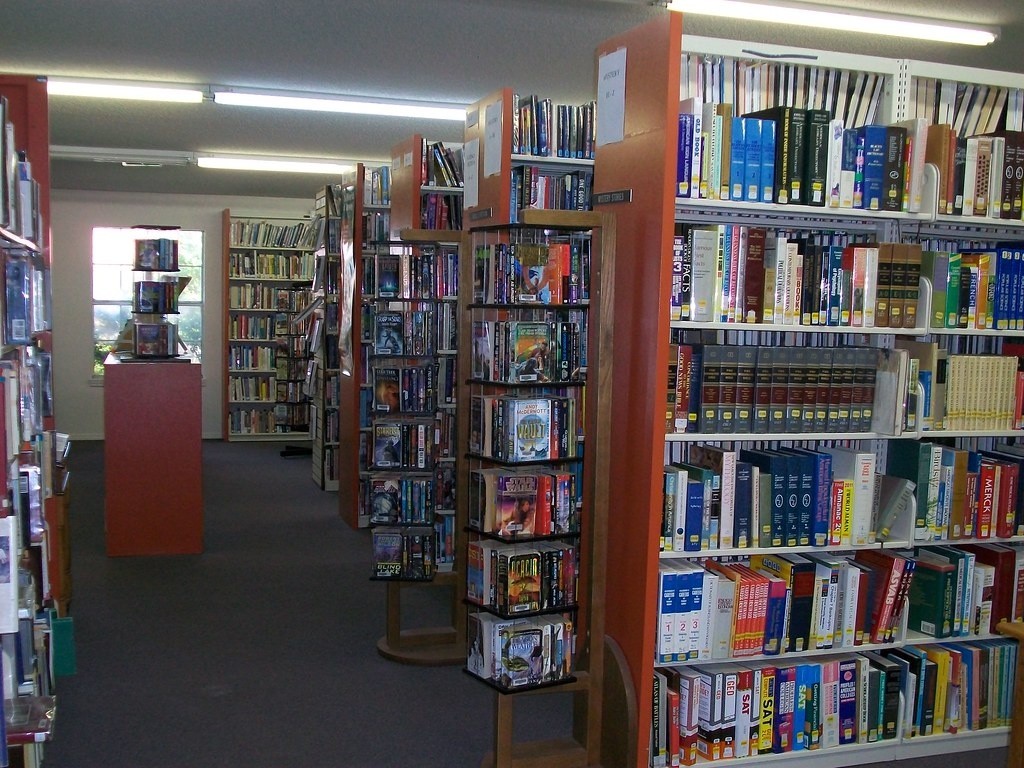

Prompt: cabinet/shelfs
[[221, 207, 312, 441], [0, 72, 61, 768], [310, 13, 1024, 768]]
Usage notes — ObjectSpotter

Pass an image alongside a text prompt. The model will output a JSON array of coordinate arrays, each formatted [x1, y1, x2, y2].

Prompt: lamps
[[209, 85, 469, 121], [648, 0, 1003, 46], [49, 144, 194, 167], [47, 76, 210, 105], [197, 152, 356, 174]]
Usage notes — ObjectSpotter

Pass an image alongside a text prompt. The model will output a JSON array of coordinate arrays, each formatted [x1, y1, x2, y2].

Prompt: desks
[[103, 350, 203, 556]]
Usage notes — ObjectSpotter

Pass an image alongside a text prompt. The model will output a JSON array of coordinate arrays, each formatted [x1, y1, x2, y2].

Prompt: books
[[226, 41, 1024, 768], [136, 238, 178, 355], [0, 97, 77, 768]]
